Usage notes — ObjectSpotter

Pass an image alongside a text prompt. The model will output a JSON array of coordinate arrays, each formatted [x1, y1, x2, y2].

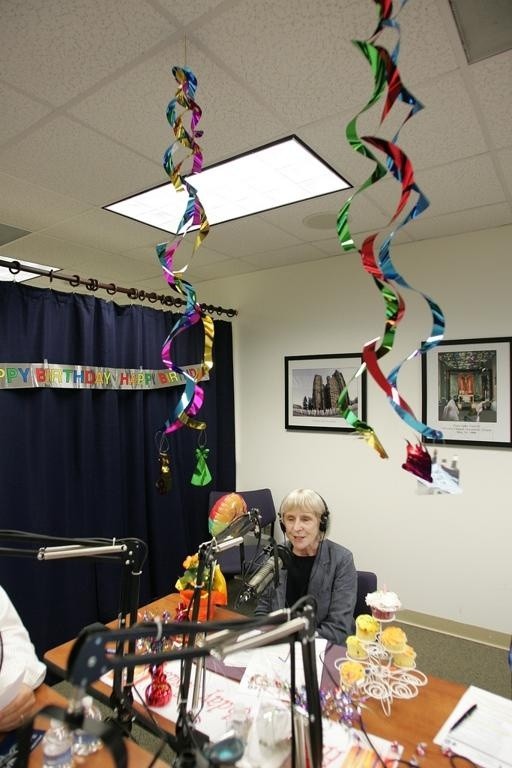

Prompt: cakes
[[339, 661, 366, 690], [394, 645, 417, 668], [345, 636, 367, 658], [355, 614, 379, 642], [365, 591, 401, 620], [381, 626, 407, 651]]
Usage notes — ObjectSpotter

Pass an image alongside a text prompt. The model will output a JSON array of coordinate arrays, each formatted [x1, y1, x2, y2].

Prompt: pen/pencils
[[448, 704, 477, 732]]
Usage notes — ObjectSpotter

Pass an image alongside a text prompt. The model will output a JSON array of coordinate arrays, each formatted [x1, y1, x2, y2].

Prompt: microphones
[[243, 545, 295, 602]]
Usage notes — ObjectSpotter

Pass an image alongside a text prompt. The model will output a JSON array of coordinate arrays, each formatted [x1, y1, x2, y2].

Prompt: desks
[[43, 592, 483, 767], [27, 680, 179, 767]]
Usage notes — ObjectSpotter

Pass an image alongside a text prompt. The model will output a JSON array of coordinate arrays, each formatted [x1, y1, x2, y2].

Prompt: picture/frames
[[284, 353, 366, 432], [421, 337, 512, 447]]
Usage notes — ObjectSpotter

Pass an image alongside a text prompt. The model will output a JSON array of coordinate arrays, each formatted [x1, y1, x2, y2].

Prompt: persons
[[254, 488, 357, 646], [0, 583, 46, 731]]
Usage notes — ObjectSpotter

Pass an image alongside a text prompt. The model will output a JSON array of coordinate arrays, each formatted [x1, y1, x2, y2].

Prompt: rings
[[19, 713, 26, 725]]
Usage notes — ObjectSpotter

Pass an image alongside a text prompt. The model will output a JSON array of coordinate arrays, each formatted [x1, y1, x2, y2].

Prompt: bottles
[[73, 696, 102, 756], [42, 719, 71, 768]]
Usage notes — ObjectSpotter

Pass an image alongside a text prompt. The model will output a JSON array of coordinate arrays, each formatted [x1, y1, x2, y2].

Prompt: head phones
[[278, 488, 330, 533]]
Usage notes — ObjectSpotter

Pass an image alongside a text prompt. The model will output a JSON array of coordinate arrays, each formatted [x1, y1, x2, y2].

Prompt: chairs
[[353, 571, 378, 620], [207, 489, 276, 576]]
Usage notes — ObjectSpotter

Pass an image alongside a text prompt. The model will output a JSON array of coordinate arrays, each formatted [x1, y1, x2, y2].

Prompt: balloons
[[209, 493, 247, 538]]
